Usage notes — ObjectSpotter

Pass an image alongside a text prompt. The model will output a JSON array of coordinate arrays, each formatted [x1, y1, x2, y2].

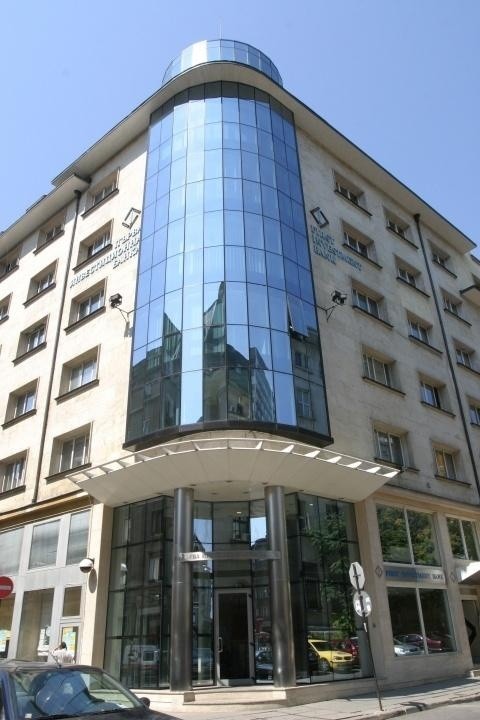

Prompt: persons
[[51, 641, 73, 665]]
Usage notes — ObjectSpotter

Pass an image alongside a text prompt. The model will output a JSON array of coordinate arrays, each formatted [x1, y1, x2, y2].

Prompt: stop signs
[[0, 576, 13, 600]]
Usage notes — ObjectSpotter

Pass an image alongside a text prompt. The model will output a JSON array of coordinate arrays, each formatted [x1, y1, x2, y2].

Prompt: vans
[[123, 646, 159, 681]]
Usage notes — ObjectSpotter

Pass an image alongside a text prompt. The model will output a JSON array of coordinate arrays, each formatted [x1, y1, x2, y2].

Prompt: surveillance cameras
[[78, 559, 94, 573]]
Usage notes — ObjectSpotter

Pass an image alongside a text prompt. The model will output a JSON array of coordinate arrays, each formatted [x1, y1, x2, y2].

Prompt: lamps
[[108, 293, 128, 322], [326, 289, 347, 321]]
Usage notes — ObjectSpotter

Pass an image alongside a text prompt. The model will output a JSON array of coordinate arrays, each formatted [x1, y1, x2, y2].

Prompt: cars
[[393, 630, 451, 656], [0, 657, 182, 720], [193, 649, 216, 677], [253, 640, 359, 681]]
